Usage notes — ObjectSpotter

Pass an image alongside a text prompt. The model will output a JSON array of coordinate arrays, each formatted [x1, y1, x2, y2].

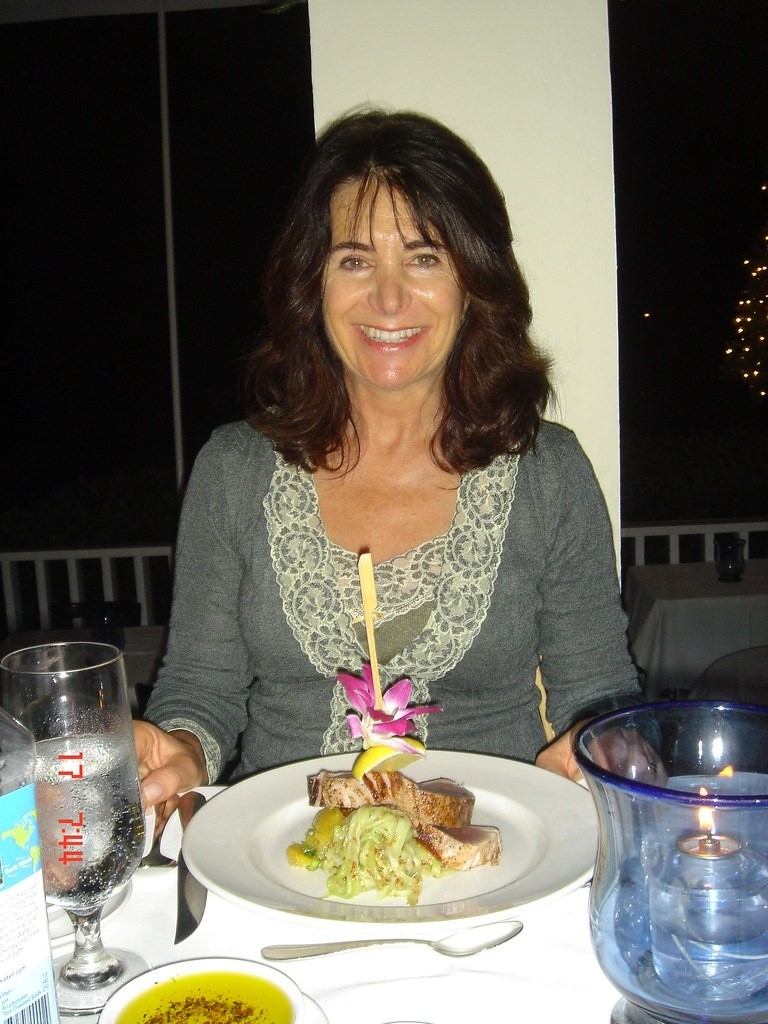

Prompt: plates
[[182, 753, 601, 934], [302, 993, 330, 1024]]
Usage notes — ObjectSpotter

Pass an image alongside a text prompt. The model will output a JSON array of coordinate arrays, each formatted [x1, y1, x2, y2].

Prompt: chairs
[[52, 601, 141, 629], [672, 646, 768, 771]]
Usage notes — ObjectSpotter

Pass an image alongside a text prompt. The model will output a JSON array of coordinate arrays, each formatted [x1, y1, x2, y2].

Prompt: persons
[[121, 108, 668, 810]]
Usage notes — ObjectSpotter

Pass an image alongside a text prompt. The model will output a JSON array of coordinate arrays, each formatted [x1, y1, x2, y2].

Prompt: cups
[[88, 601, 124, 651], [713, 537, 747, 583], [97, 957, 305, 1024], [573, 699, 768, 1024]]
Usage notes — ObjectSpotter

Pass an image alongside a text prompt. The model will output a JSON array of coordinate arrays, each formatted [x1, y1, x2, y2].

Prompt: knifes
[[174, 791, 207, 946]]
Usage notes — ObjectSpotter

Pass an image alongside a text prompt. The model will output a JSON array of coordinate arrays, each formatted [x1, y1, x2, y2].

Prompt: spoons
[[261, 920, 525, 961], [136, 793, 179, 869]]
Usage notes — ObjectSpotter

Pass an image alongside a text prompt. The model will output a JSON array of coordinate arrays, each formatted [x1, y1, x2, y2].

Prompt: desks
[[628, 559, 768, 700], [0, 626, 165, 684]]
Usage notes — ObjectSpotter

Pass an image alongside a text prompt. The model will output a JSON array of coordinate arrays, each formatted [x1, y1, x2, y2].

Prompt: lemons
[[351, 737, 426, 781]]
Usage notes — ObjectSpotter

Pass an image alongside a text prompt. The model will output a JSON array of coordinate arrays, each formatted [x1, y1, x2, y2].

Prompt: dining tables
[[57, 786, 622, 1024]]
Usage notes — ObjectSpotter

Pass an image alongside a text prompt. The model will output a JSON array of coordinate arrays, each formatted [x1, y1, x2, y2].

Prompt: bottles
[[0, 702, 62, 1024]]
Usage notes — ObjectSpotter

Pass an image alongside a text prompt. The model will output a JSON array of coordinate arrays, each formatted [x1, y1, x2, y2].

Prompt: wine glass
[[1, 639, 153, 1016]]
